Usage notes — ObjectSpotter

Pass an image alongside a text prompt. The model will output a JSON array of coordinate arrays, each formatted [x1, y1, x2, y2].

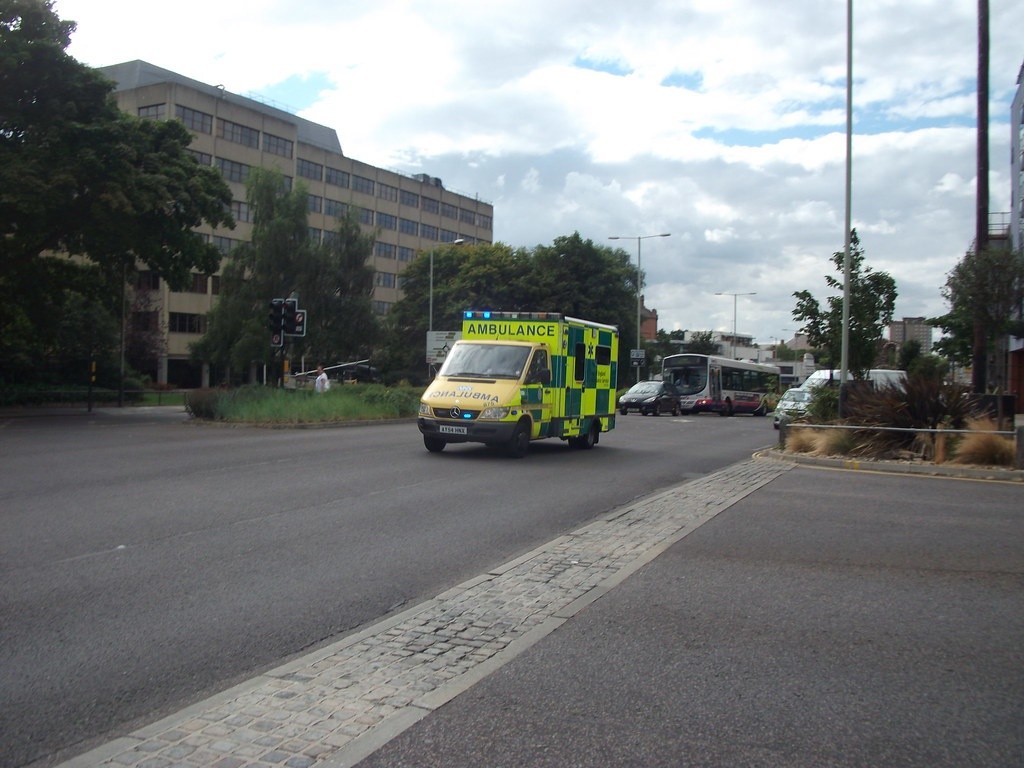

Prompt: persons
[[514, 355, 531, 376], [674, 374, 686, 386], [316, 366, 330, 393]]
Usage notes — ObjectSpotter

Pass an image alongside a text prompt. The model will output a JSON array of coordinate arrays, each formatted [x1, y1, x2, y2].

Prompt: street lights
[[608, 234, 671, 383], [714, 292, 757, 360], [783, 328, 797, 388], [769, 336, 777, 363], [430, 239, 464, 331]]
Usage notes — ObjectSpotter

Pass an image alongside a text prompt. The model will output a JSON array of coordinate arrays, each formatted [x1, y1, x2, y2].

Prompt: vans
[[773, 370, 909, 430]]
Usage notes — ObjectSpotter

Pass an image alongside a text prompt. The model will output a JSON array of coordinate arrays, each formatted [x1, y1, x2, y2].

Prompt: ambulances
[[417, 312, 619, 458]]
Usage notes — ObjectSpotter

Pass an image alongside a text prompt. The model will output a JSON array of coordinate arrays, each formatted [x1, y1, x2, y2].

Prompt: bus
[[661, 354, 781, 416]]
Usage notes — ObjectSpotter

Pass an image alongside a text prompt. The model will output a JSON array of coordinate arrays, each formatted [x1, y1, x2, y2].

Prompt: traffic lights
[[283, 299, 297, 334], [268, 299, 284, 335]]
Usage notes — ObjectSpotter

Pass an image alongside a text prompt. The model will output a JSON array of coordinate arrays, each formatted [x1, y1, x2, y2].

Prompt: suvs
[[620, 379, 681, 416]]
